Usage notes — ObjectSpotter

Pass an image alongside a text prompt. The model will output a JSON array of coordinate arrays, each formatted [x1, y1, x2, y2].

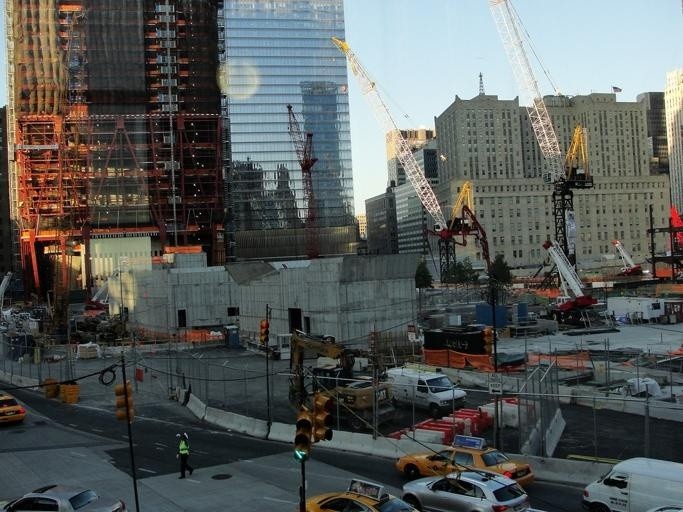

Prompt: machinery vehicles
[[286, 329, 396, 433], [541, 241, 598, 327]]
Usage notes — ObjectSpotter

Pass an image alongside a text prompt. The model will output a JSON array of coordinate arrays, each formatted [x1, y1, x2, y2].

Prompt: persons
[[174, 431, 194, 479]]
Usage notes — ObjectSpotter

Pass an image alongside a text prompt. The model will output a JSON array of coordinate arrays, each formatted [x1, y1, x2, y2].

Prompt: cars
[[400, 469, 530, 512], [0, 393, 26, 425], [394, 434, 533, 488], [0, 482, 128, 512], [645, 504, 682, 512], [292, 478, 419, 512]]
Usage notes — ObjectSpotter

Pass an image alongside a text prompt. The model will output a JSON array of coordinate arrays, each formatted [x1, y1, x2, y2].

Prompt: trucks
[[578, 458, 682, 512], [382, 367, 467, 415]]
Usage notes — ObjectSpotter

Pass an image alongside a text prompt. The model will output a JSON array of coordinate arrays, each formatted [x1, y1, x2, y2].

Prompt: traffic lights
[[311, 391, 334, 445], [293, 410, 312, 466], [114, 379, 135, 421], [259, 318, 269, 343]]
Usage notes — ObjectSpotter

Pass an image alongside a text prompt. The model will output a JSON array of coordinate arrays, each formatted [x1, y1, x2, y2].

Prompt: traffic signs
[[488, 382, 502, 395]]
